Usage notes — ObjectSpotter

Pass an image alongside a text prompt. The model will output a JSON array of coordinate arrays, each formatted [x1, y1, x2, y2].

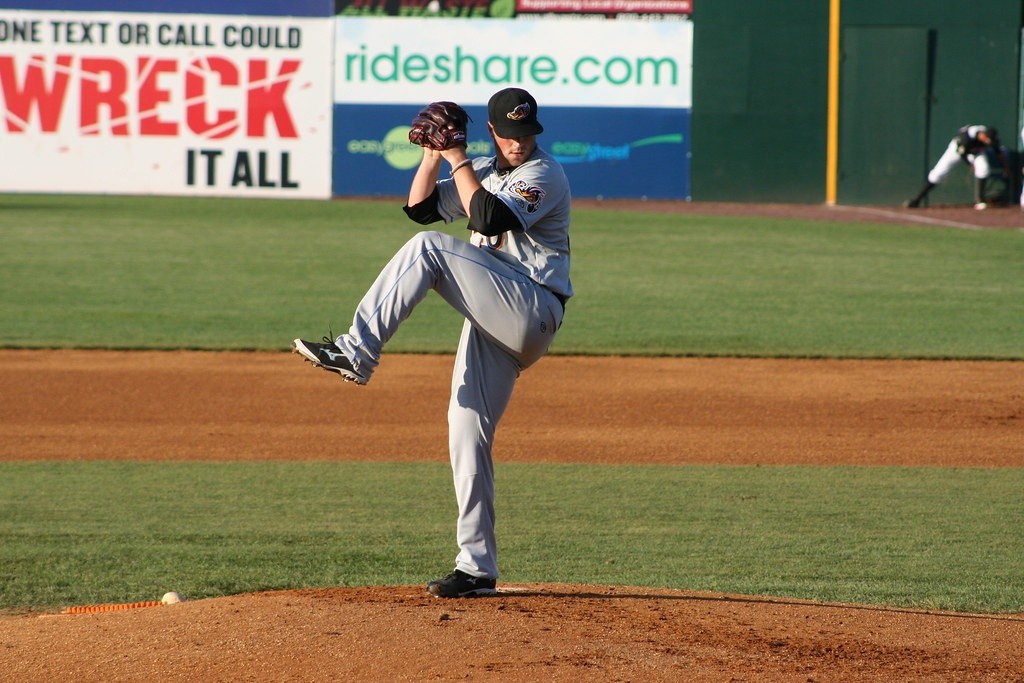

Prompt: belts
[[551, 290, 565, 315]]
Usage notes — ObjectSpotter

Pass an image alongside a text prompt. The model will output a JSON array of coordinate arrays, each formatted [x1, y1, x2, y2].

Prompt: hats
[[985, 129, 999, 147], [488, 88, 544, 139]]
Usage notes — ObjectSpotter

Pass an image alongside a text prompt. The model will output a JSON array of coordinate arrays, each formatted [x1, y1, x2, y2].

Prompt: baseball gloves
[[409, 101, 469, 151]]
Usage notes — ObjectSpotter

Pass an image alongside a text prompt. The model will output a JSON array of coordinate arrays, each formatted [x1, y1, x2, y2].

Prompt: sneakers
[[425, 569, 497, 598], [291, 326, 368, 385]]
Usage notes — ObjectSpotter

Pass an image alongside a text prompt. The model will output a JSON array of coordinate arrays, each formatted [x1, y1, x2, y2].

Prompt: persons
[[291, 86, 573, 599], [903, 123, 1024, 211]]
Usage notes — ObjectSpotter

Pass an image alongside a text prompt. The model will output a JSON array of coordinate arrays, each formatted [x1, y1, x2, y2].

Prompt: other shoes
[[973, 201, 988, 211], [904, 199, 922, 210]]
